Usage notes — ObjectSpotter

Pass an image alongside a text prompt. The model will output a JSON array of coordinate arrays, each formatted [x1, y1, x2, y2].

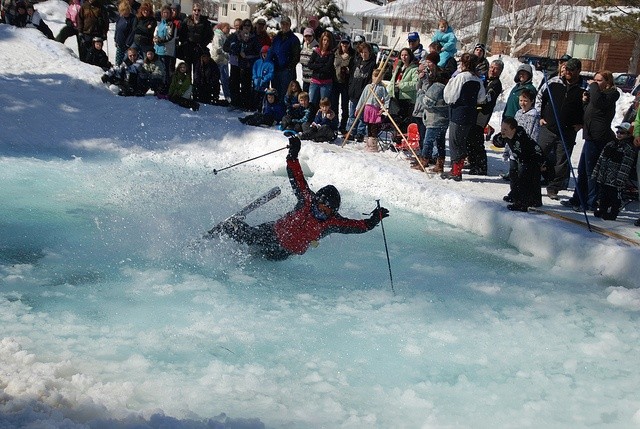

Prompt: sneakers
[[601, 212, 617, 221], [572, 205, 598, 212], [560, 199, 573, 207], [594, 210, 604, 217], [500, 172, 510, 181]]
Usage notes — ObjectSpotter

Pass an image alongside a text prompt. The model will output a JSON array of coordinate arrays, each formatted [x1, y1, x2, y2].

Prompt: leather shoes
[[547, 189, 559, 200], [507, 203, 527, 212], [503, 195, 516, 202]]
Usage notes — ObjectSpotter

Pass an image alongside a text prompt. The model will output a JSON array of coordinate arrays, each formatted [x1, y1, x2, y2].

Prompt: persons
[[77, 0, 109, 63], [307, 30, 335, 106], [632, 100, 640, 226], [135, 48, 167, 95], [211, 22, 231, 104], [472, 44, 495, 142], [412, 52, 443, 165], [499, 89, 539, 180], [114, 0, 135, 65], [620, 83, 640, 122], [85, 36, 113, 71], [476, 61, 489, 85], [178, 3, 214, 95], [231, 19, 261, 104], [238, 88, 282, 127], [500, 64, 538, 180], [194, 47, 220, 103], [536, 62, 570, 115], [223, 18, 243, 110], [281, 80, 301, 129], [133, 0, 157, 55], [55, 0, 84, 44], [460, 60, 503, 169], [560, 69, 620, 214], [2, 0, 46, 34], [349, 35, 365, 68], [170, 3, 187, 29], [224, 136, 390, 262], [153, 4, 178, 83], [13, 3, 27, 27], [407, 32, 429, 63], [167, 62, 201, 112], [440, 53, 486, 181], [430, 41, 441, 52], [591, 122, 635, 221], [538, 54, 572, 91], [251, 45, 275, 112], [285, 91, 314, 130], [412, 68, 447, 173], [354, 68, 390, 146], [540, 58, 584, 201], [237, 29, 251, 57], [345, 42, 376, 141], [300, 27, 319, 90], [296, 96, 337, 142], [387, 48, 420, 142], [102, 47, 143, 96], [331, 36, 352, 134], [413, 58, 429, 149], [272, 16, 301, 100], [430, 18, 457, 66], [500, 117, 545, 212], [256, 18, 271, 45]]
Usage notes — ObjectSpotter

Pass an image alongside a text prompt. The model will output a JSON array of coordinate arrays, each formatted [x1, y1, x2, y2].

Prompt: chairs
[[394, 123, 421, 159], [378, 113, 397, 151]]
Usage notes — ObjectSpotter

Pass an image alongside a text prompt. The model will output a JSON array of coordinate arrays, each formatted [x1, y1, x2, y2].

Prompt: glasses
[[616, 131, 624, 135], [243, 24, 250, 27], [317, 203, 332, 216], [193, 9, 200, 12], [475, 44, 485, 49]]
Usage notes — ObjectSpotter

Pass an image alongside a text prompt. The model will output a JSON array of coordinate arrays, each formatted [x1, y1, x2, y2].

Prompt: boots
[[429, 156, 445, 172], [367, 137, 378, 152], [411, 156, 428, 172], [441, 162, 462, 181], [459, 159, 465, 169]]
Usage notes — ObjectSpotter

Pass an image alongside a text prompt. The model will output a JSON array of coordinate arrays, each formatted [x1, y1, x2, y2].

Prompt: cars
[[373, 45, 401, 80], [614, 72, 636, 92]]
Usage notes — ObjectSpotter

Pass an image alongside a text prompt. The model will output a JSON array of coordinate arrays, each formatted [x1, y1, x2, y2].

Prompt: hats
[[408, 32, 419, 41], [615, 123, 634, 134], [558, 55, 572, 61], [425, 53, 440, 64], [353, 36, 365, 45], [312, 184, 341, 222], [564, 59, 581, 71], [280, 16, 291, 25], [257, 19, 266, 25], [339, 36, 351, 44], [303, 28, 314, 36]]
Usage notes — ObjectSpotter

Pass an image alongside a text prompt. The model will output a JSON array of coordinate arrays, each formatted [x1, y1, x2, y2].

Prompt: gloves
[[283, 134, 302, 161], [364, 207, 389, 231]]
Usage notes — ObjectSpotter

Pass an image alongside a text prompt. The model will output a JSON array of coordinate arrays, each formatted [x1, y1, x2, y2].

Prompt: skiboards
[[528, 207, 640, 245], [203, 186, 280, 239]]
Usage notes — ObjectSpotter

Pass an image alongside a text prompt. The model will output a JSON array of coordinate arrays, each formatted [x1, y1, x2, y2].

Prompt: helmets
[[91, 37, 104, 48], [493, 132, 506, 148]]
